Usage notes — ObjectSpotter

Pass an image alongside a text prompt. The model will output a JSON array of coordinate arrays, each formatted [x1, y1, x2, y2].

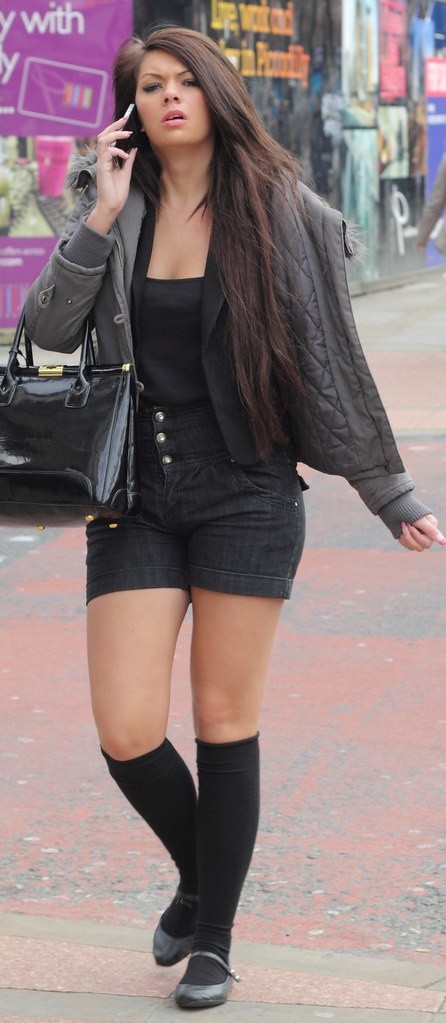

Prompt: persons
[[416, 155, 446, 256], [66, 134, 95, 219], [6, 133, 36, 213], [23, 26, 446, 1009]]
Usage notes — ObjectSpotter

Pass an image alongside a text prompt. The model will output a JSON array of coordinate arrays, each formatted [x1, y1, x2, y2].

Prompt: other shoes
[[174, 951, 240, 1005], [153, 888, 200, 967]]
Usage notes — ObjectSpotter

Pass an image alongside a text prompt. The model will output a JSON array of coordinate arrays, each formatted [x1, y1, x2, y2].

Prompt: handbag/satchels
[[0, 305, 142, 518]]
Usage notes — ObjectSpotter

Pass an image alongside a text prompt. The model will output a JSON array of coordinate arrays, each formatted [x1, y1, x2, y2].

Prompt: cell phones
[[112, 104, 142, 169]]
